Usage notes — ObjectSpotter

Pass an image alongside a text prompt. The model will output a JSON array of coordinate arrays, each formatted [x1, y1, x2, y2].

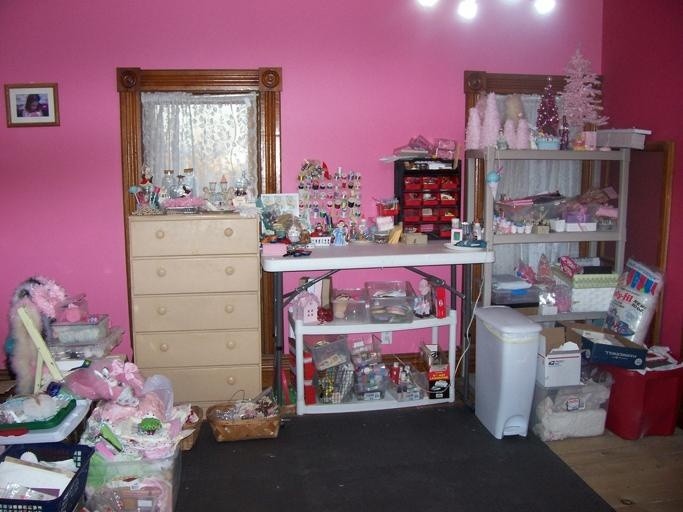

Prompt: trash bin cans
[[474, 305, 543, 440]]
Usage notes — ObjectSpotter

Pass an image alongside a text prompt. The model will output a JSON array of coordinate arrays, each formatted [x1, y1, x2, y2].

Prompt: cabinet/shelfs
[[286, 308, 457, 416], [465, 134, 644, 322], [260, 239, 495, 416], [126, 212, 262, 420]]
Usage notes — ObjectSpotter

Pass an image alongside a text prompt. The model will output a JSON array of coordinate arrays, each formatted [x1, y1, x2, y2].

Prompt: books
[[491, 274, 532, 290], [299, 277, 332, 308]]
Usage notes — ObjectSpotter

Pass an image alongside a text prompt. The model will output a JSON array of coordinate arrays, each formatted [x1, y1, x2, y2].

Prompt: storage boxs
[[529, 364, 615, 441], [535, 327, 584, 388], [601, 364, 682, 440], [0, 400, 93, 445], [554, 321, 647, 370], [83, 443, 182, 511]]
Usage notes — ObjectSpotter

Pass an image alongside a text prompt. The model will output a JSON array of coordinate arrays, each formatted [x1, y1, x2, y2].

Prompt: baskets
[[181, 400, 282, 451], [0, 441, 96, 512], [318, 369, 353, 404]]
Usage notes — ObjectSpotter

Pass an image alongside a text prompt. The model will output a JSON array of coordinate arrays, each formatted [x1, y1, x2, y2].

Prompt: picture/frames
[[5, 82, 59, 128]]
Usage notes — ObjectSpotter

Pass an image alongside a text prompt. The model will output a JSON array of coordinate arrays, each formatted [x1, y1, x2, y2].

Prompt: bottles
[[560, 115, 570, 151], [165, 169, 195, 199], [497, 130, 508, 150], [237, 169, 251, 196], [461, 222, 482, 241], [391, 362, 410, 392]]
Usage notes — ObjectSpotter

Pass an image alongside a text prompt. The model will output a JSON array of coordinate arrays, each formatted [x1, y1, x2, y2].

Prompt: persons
[[334, 220, 348, 247], [22, 94, 45, 118]]
